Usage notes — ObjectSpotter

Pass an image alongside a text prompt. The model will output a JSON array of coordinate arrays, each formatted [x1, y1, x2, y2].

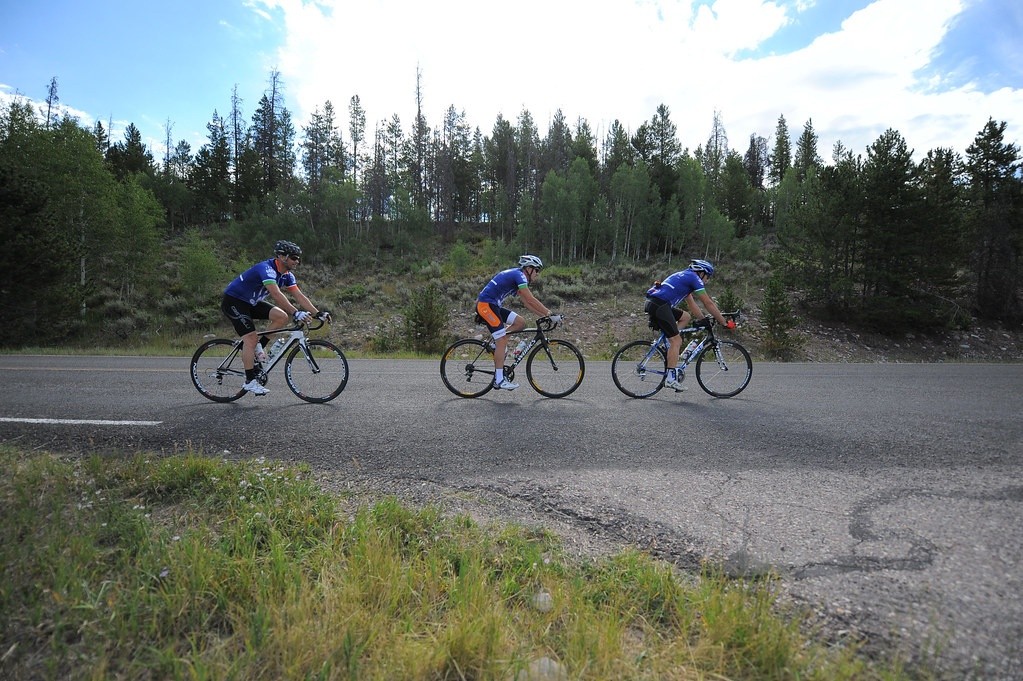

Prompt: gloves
[[294, 311, 313, 325], [549, 312, 563, 325], [724, 319, 741, 329], [316, 311, 332, 324]]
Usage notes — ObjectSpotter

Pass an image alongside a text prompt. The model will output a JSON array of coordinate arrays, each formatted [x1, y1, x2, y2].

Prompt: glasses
[[534, 268, 541, 274], [290, 254, 300, 261]]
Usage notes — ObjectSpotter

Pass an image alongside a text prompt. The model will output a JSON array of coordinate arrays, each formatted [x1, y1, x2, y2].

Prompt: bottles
[[681, 339, 698, 360], [266, 338, 285, 360], [255, 341, 265, 362], [513, 338, 528, 355]]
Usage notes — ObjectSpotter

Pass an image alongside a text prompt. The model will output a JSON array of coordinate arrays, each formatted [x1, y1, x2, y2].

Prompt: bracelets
[[546, 309, 552, 317], [723, 320, 729, 327]]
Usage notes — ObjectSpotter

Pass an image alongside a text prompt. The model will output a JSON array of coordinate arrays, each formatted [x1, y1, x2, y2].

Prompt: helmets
[[689, 257, 714, 278], [518, 254, 543, 270], [273, 239, 302, 255]]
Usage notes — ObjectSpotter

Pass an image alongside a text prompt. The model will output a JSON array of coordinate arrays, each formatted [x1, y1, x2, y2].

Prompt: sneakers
[[493, 380, 519, 390], [663, 379, 688, 391], [490, 342, 506, 357], [662, 341, 671, 350], [254, 343, 266, 363], [243, 379, 270, 394]]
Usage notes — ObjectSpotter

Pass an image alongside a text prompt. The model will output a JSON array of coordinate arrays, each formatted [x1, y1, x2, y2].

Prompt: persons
[[643, 258, 735, 390], [221, 239, 332, 394], [475, 254, 564, 390]]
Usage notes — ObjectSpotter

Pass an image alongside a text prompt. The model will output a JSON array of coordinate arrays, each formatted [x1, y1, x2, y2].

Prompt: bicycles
[[611, 308, 753, 399], [189, 315, 350, 405], [439, 311, 586, 400]]
